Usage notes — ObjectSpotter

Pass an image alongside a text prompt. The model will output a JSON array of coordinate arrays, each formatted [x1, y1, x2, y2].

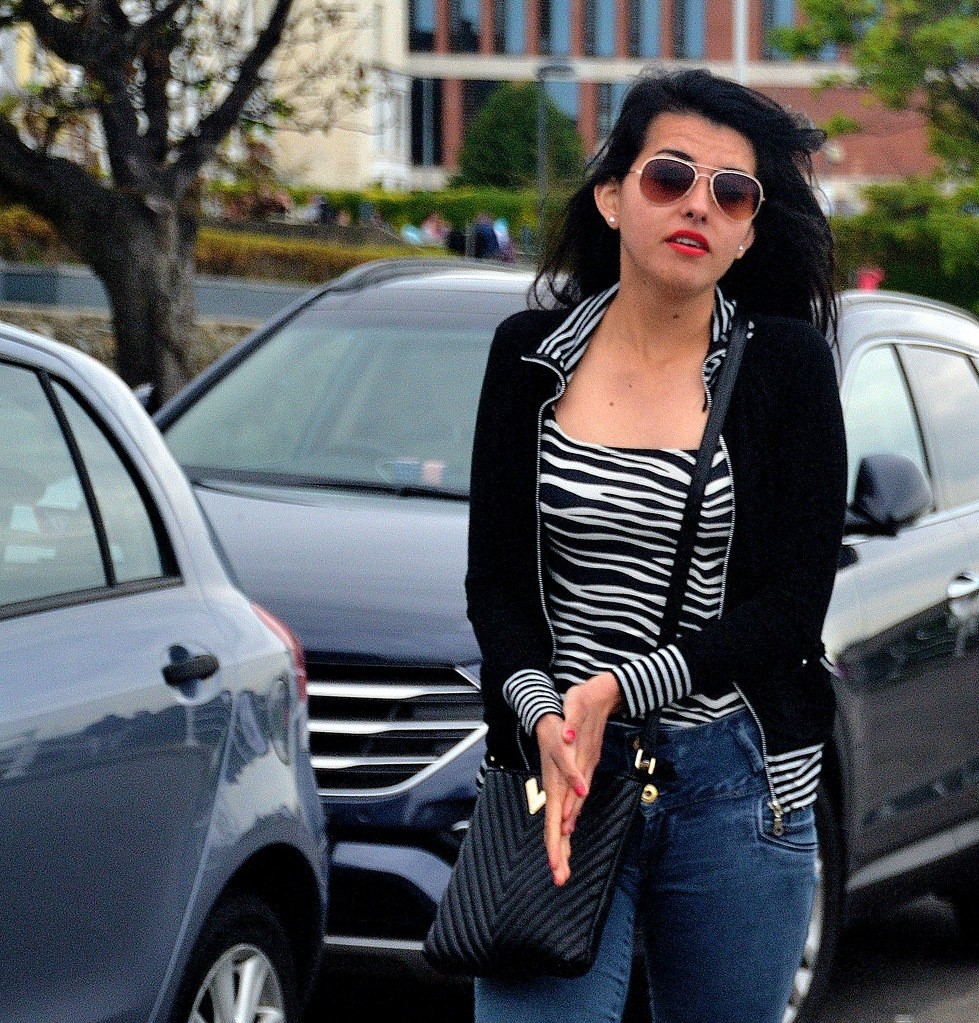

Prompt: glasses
[[628, 155, 765, 224]]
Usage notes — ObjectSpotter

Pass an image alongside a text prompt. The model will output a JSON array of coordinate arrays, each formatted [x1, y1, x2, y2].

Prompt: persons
[[460, 71, 846, 1023], [450, 211, 511, 258], [209, 186, 384, 234], [420, 209, 445, 245]]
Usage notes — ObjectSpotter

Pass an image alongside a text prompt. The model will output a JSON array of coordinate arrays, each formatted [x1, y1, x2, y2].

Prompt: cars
[[150, 257, 979, 991], [0, 322, 333, 1023]]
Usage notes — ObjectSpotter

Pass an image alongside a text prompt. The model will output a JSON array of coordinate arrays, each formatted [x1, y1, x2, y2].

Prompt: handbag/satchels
[[421, 737, 659, 975]]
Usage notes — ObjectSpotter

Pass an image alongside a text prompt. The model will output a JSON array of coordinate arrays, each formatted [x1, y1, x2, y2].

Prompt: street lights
[[537, 63, 574, 242]]
